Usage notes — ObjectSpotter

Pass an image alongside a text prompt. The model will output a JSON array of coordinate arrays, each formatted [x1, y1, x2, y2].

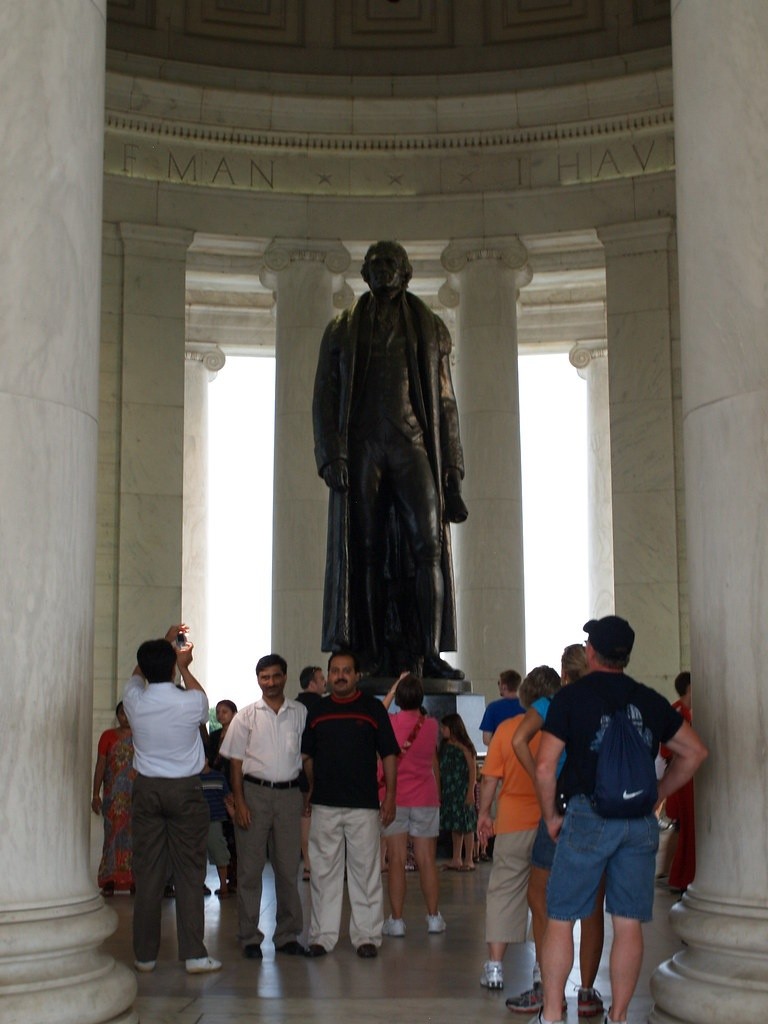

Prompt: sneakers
[[427, 911, 446, 932], [135, 960, 156, 971], [506, 982, 567, 1013], [574, 984, 603, 1016], [532, 962, 541, 983], [481, 960, 504, 989], [382, 914, 406, 936], [600, 1006, 626, 1023], [186, 957, 222, 973], [528, 1005, 563, 1024]]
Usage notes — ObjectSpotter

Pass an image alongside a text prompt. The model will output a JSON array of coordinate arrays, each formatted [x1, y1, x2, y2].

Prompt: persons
[[313, 239, 469, 681], [196, 701, 242, 900], [374, 669, 476, 937], [476, 615, 709, 1024], [219, 648, 402, 959], [92, 701, 135, 895], [122, 623, 222, 974]]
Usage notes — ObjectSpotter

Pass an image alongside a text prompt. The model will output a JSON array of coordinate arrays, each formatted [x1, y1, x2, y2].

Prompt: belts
[[244, 775, 298, 789]]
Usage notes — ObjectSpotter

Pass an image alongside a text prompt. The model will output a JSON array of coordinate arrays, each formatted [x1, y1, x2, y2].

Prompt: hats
[[583, 615, 634, 650]]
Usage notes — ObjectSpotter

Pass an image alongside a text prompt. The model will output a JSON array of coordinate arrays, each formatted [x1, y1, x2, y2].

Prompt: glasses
[[583, 641, 588, 647], [498, 681, 500, 685]]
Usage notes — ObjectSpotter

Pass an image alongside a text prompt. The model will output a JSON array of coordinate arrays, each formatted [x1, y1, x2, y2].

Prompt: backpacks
[[593, 709, 658, 819]]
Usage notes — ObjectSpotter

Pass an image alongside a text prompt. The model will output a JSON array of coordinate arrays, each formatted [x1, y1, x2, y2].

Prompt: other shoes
[[306, 945, 325, 956], [358, 944, 376, 958], [244, 945, 262, 959], [276, 941, 305, 955], [99, 850, 491, 897]]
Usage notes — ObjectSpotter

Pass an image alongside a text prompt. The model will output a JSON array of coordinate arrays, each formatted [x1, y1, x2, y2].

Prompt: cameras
[[176, 633, 187, 647]]
